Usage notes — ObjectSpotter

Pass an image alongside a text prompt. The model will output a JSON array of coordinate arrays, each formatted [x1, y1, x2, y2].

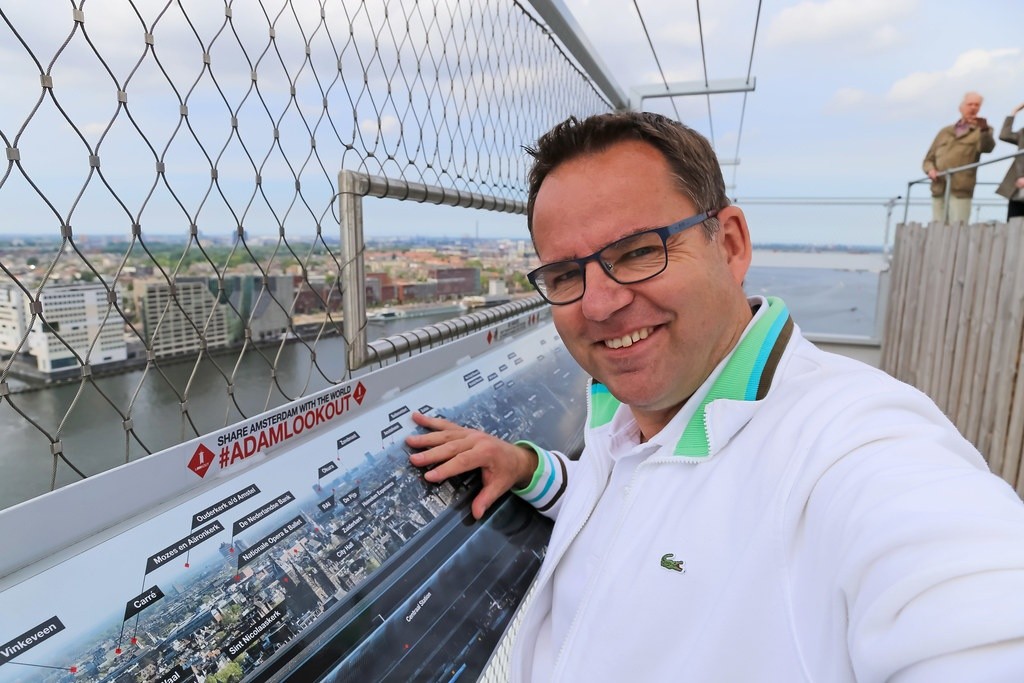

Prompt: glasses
[[526, 203, 727, 305]]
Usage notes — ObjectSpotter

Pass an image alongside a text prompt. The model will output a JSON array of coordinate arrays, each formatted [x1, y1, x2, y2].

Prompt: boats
[[261, 325, 340, 343], [366, 308, 406, 323], [461, 278, 512, 309]]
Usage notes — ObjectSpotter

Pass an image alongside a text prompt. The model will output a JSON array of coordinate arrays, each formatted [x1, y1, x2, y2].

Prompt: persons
[[920, 92, 996, 225], [405, 107, 1024, 683], [995, 99, 1024, 222]]
